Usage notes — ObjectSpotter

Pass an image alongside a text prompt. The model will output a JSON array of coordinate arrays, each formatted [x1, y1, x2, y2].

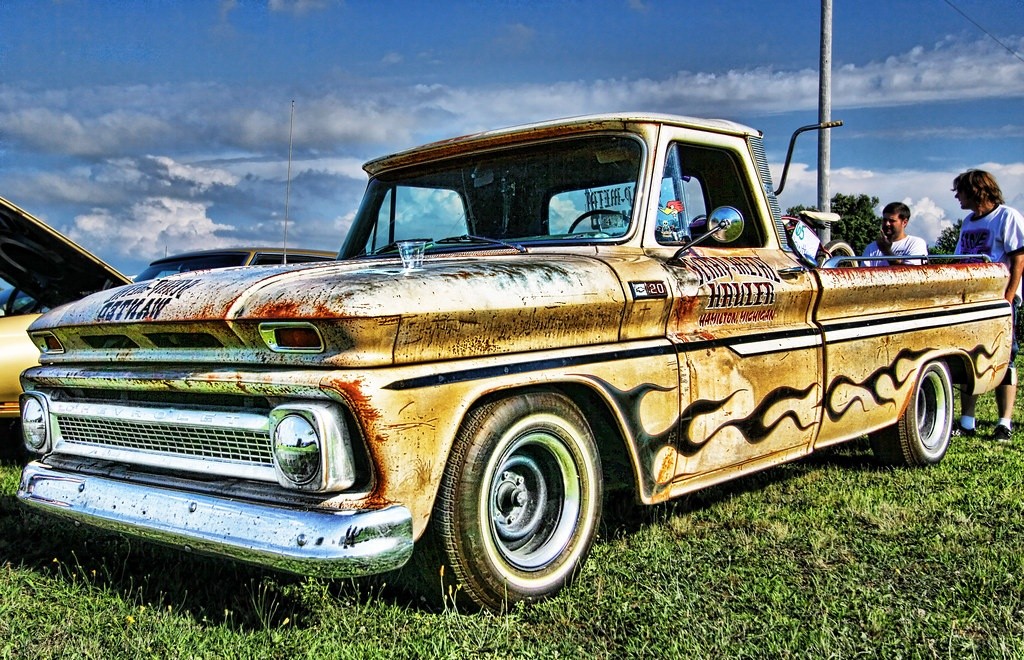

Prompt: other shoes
[[950, 421, 976, 438], [993, 425, 1011, 442]]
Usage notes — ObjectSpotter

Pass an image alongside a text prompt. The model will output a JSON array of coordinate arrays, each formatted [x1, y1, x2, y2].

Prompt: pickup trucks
[[16, 112, 1011, 618]]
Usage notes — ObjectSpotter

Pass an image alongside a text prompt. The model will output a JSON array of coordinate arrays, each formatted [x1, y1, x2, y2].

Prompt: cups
[[397, 241, 426, 268]]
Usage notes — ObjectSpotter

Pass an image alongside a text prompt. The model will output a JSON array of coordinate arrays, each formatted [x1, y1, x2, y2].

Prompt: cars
[[0, 196, 339, 470]]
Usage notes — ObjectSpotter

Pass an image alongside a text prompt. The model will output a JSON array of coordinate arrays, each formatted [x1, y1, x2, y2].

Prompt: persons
[[949, 169, 1024, 443], [860, 202, 929, 268]]
